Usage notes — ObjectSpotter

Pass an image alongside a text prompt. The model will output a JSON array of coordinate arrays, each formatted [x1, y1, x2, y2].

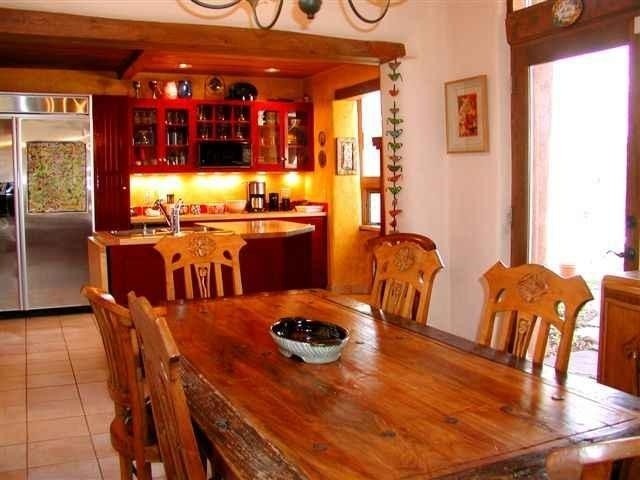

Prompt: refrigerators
[[0, 91, 96, 315]]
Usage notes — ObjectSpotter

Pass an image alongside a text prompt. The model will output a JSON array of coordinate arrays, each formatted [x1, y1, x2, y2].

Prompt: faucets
[[152, 198, 184, 232]]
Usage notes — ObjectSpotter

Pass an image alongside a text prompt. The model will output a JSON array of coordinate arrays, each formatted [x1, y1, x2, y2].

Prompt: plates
[[268, 317, 348, 363]]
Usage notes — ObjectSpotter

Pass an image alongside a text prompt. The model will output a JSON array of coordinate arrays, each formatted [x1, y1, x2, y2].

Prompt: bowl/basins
[[207, 203, 224, 213], [295, 205, 324, 213], [226, 199, 247, 212]]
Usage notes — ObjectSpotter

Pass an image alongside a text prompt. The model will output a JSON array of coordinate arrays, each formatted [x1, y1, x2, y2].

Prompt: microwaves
[[199, 142, 250, 166]]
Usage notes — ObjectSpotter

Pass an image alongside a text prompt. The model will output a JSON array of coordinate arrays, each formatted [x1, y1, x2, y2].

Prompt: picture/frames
[[443, 74, 489, 152], [335, 136, 356, 178]]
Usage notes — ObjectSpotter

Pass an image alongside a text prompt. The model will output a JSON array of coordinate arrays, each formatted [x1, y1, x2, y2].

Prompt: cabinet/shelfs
[[128, 98, 315, 175], [597, 273, 640, 394]]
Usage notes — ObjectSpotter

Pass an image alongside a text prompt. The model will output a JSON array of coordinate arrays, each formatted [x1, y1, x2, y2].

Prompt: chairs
[[546, 435, 639, 479], [363, 233, 434, 317], [153, 231, 247, 300], [127, 288, 208, 480], [369, 241, 443, 324], [78, 284, 165, 480], [476, 261, 593, 372]]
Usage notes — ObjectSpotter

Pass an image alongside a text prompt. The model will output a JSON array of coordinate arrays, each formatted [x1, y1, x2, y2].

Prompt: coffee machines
[[247, 181, 267, 212]]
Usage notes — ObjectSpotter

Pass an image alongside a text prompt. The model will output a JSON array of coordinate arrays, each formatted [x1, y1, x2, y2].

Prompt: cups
[[283, 198, 290, 207], [269, 193, 279, 207]]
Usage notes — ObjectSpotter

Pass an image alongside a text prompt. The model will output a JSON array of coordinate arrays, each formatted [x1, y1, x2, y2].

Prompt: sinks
[[109, 226, 169, 238], [166, 226, 221, 234]]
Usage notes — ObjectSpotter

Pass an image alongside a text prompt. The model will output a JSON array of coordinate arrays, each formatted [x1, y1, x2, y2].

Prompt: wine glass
[[133, 80, 141, 98], [148, 80, 157, 99], [134, 110, 153, 124]]
